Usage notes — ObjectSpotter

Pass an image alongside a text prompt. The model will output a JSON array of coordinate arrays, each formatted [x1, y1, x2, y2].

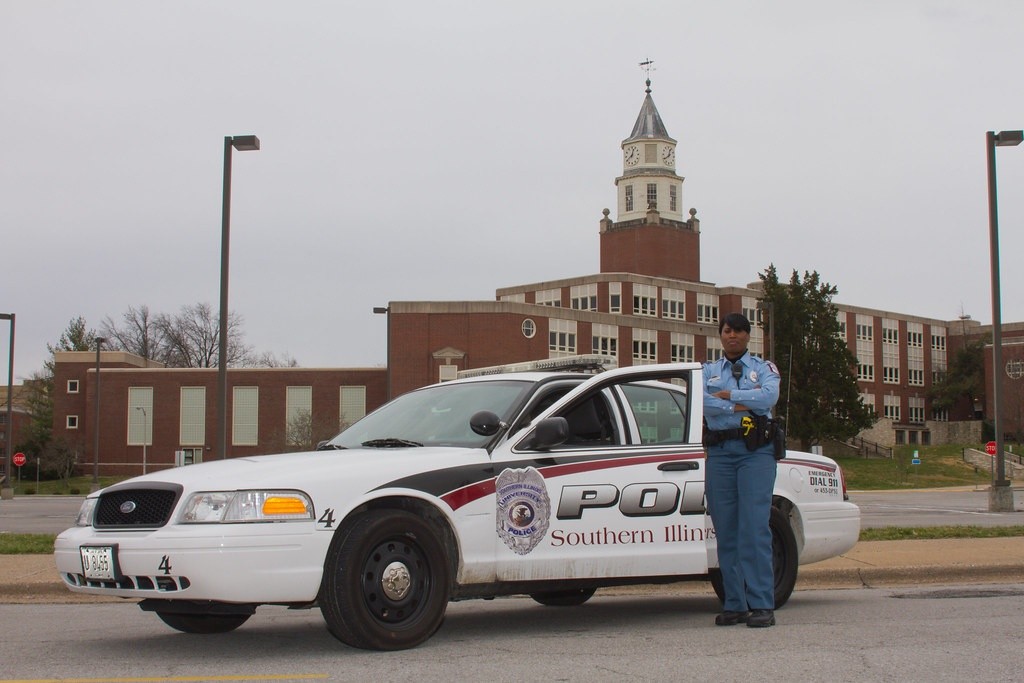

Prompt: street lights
[[216, 135, 261, 460], [0, 313, 15, 500], [986, 128, 1024, 513], [90, 337, 109, 495]]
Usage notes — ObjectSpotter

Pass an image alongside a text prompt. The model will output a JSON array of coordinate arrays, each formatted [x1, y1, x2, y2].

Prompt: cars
[[53, 355, 862, 652]]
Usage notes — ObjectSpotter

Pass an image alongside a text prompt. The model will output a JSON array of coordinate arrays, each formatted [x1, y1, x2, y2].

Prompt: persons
[[701, 312, 782, 628]]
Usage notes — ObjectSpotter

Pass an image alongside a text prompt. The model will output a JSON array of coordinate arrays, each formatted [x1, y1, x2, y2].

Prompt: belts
[[716, 428, 743, 440]]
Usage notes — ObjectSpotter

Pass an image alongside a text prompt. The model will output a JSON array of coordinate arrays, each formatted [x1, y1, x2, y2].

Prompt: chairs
[[567, 399, 611, 447]]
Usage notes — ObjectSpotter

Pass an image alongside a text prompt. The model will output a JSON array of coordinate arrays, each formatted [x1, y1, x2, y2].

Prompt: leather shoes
[[715, 610, 749, 626], [746, 608, 776, 628]]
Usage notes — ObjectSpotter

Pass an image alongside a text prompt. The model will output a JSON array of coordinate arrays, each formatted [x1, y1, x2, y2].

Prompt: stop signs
[[12, 452, 26, 467], [985, 440, 997, 456]]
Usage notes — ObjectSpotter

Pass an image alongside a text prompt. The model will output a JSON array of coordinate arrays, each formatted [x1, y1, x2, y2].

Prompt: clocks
[[625, 145, 640, 166], [661, 145, 676, 166]]
